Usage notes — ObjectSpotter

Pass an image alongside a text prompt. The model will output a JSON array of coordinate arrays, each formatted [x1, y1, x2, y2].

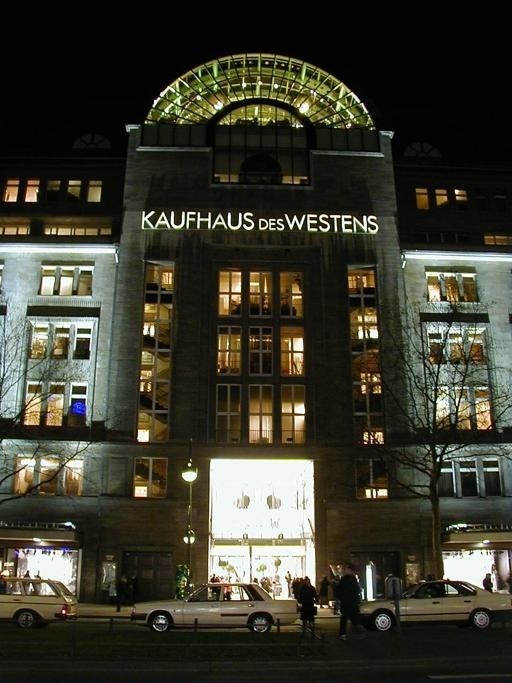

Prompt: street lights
[[179, 437, 200, 595]]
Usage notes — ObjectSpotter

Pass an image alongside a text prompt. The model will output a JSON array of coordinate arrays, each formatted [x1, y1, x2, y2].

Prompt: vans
[[0, 575, 80, 629]]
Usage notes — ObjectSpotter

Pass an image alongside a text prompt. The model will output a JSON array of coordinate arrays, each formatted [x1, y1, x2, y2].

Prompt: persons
[[0, 566, 9, 577], [34, 570, 41, 591], [492, 565, 499, 591], [206, 565, 445, 639], [483, 574, 492, 592], [507, 574, 512, 594], [109, 576, 134, 606], [23, 570, 30, 593], [9, 567, 14, 576]]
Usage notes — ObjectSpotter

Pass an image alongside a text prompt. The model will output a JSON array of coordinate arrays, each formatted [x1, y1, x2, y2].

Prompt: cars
[[356, 577, 512, 631], [130, 581, 301, 633]]
